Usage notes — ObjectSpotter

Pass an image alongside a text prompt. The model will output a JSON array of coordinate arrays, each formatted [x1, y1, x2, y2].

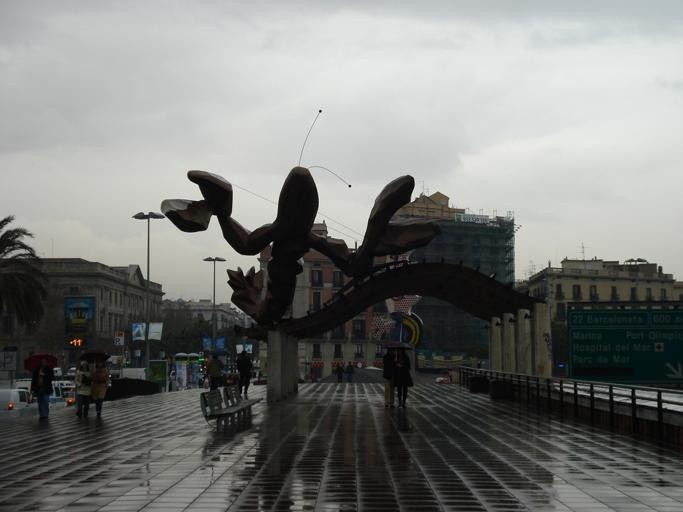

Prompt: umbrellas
[[73, 346, 110, 371], [23, 351, 58, 373], [204, 348, 232, 356], [377, 338, 415, 350]]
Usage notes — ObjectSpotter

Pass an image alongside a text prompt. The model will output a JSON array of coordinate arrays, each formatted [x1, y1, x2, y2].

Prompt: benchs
[[200, 385, 263, 433]]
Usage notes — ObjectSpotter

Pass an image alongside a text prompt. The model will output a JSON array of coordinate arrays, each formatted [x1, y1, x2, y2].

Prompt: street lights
[[132, 212, 165, 378], [203, 257, 227, 355]]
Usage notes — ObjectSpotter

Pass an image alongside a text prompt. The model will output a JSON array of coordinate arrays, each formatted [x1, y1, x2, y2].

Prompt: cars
[[1, 366, 79, 410]]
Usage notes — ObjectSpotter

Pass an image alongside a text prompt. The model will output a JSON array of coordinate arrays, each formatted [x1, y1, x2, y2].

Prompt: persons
[[205, 355, 225, 391], [345, 360, 354, 383], [334, 363, 346, 383], [395, 346, 415, 409], [90, 358, 108, 416], [381, 349, 399, 409], [28, 358, 54, 421], [235, 350, 253, 396], [73, 359, 92, 419]]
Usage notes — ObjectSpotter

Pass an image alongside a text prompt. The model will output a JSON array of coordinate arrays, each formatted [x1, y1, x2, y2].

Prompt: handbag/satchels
[[95, 370, 107, 383], [80, 373, 92, 385]]
